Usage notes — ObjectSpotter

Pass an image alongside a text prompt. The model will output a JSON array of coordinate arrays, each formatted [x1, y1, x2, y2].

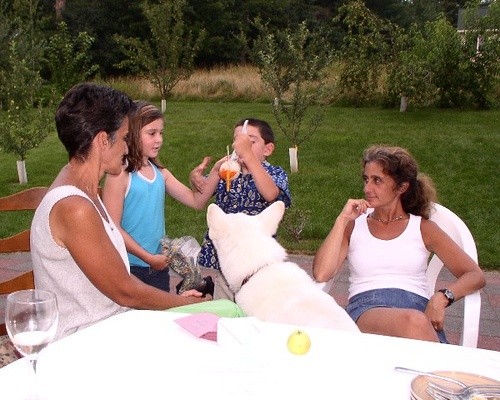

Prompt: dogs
[[206, 200, 360, 334]]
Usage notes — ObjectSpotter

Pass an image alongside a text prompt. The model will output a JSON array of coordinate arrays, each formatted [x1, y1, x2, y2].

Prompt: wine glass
[[5, 290, 59, 388]]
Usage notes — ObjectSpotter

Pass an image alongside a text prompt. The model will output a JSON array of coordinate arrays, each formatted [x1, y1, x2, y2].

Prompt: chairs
[[315, 201, 482, 349], [1, 185, 104, 368]]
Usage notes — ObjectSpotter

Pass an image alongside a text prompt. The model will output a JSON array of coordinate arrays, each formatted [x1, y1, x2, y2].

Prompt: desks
[[0, 309, 500, 400]]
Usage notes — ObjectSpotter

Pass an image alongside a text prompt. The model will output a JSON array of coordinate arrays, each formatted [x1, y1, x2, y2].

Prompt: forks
[[425, 381, 500, 400]]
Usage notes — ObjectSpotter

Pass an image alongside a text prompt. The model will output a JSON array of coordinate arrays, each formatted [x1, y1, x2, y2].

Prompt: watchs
[[437, 288, 455, 308]]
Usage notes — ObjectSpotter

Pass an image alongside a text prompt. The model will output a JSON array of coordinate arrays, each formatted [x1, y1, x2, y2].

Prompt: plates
[[410, 371, 500, 400]]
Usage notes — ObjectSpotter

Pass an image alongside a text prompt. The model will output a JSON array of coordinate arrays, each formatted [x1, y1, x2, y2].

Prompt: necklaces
[[67, 162, 98, 199], [370, 212, 403, 224]]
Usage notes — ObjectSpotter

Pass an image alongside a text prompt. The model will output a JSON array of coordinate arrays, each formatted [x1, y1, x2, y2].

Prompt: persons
[[30, 83, 213, 342], [313, 143, 486, 345], [101, 100, 232, 293], [189, 118, 293, 275]]
